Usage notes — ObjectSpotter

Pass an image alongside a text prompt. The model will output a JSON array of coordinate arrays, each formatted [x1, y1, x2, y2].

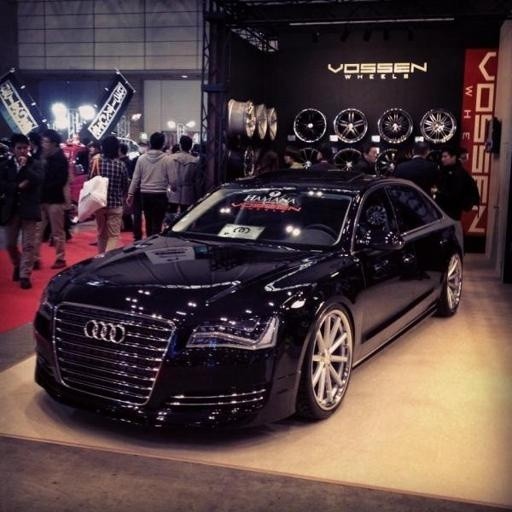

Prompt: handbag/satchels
[[78, 176, 109, 222]]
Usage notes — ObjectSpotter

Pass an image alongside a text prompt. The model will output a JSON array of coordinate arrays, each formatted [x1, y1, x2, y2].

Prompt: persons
[[287, 153, 305, 170], [0, 129, 205, 289], [393, 141, 480, 221], [253, 151, 279, 176], [352, 143, 381, 176]]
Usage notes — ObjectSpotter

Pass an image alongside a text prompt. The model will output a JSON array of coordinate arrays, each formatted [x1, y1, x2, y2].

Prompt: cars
[[60, 141, 91, 218], [115, 136, 142, 160], [32, 167, 465, 444]]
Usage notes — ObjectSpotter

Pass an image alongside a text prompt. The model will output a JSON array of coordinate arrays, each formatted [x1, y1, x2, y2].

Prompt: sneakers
[[52, 258, 66, 269], [32, 261, 39, 270], [13, 267, 20, 282], [21, 278, 31, 289]]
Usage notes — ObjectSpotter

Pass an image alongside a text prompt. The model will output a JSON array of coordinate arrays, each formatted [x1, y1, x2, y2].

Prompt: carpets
[[1, 223, 158, 332]]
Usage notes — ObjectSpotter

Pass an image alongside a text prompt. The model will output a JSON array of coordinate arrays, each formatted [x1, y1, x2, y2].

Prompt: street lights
[[48, 101, 97, 141], [167, 119, 197, 144]]
